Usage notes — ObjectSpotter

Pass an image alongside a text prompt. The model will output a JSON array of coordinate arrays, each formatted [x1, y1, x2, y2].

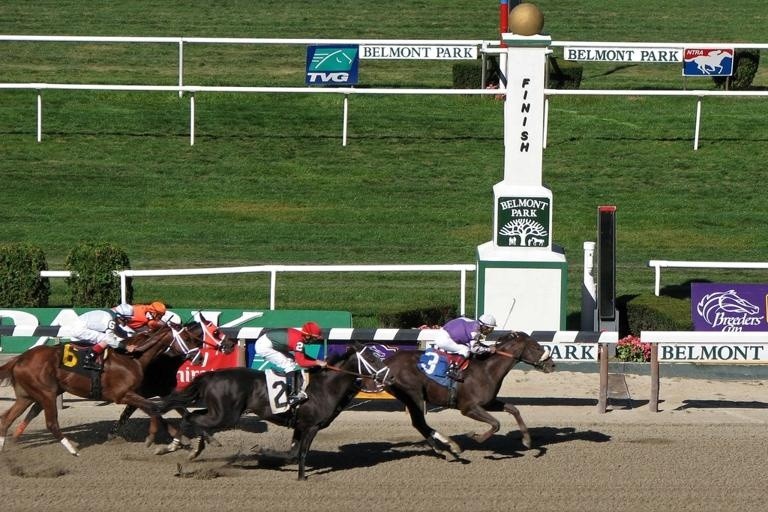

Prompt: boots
[[83, 348, 102, 370], [445, 355, 464, 382], [286, 371, 303, 405]]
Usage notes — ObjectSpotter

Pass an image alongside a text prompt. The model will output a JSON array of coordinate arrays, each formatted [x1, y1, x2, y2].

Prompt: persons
[[110, 301, 168, 330], [433, 314, 497, 382], [253, 322, 329, 409], [63, 303, 135, 368]]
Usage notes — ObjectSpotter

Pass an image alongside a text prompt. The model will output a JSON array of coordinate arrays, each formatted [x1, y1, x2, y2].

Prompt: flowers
[[615, 335, 651, 362]]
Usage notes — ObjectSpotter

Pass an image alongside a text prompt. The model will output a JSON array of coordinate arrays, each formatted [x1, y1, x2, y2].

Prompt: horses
[[150, 340, 396, 481], [382, 331, 556, 462], [0, 319, 204, 457], [11, 312, 235, 445]]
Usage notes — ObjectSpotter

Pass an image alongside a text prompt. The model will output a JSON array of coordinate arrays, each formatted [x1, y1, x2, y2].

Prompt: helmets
[[114, 304, 134, 320], [301, 322, 321, 337], [149, 302, 165, 315], [479, 314, 496, 328]]
